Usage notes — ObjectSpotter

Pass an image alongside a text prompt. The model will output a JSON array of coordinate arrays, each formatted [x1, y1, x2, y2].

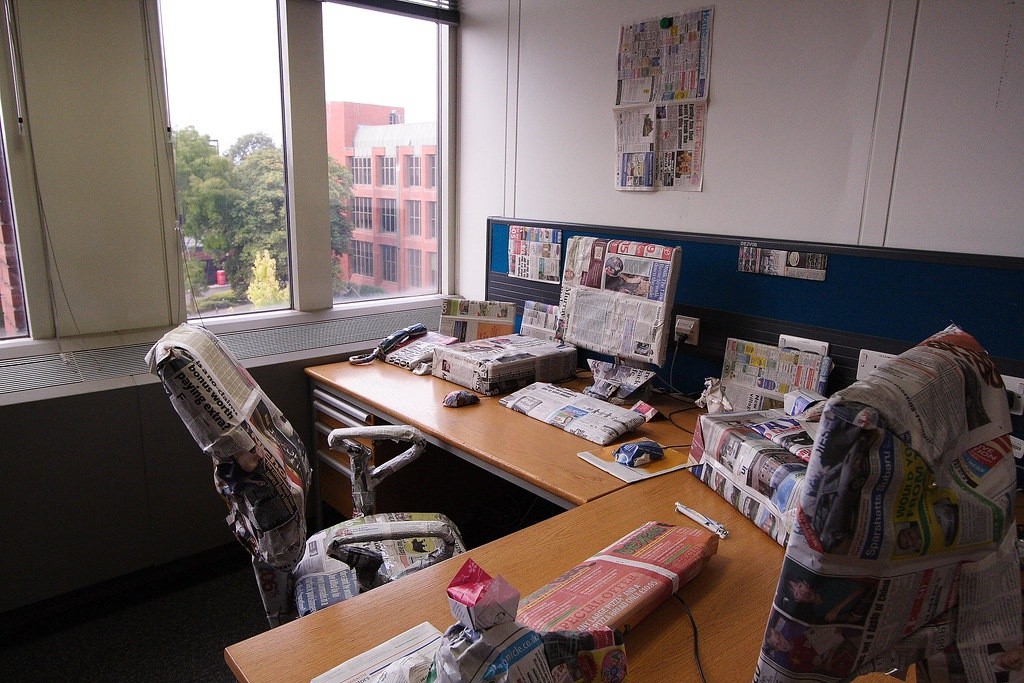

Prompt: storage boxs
[[432, 333, 579, 397], [683, 406, 808, 547]]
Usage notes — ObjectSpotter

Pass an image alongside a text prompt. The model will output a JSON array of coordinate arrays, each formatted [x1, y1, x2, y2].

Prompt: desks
[[219, 360, 1024, 683]]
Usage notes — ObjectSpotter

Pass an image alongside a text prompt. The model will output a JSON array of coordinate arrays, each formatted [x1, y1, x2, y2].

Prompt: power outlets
[[673, 315, 700, 346]]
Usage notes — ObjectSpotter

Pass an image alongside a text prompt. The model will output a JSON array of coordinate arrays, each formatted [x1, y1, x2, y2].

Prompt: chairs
[[142, 322, 468, 628]]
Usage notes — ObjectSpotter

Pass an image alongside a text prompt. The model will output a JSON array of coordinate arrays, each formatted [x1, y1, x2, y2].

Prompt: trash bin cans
[[217, 270, 226, 285]]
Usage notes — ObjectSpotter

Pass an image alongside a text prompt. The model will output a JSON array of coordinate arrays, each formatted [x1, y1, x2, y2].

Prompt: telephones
[[374, 324, 459, 371]]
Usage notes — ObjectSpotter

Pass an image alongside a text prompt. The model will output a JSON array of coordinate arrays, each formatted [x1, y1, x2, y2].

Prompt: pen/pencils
[[674, 501, 729, 540]]
